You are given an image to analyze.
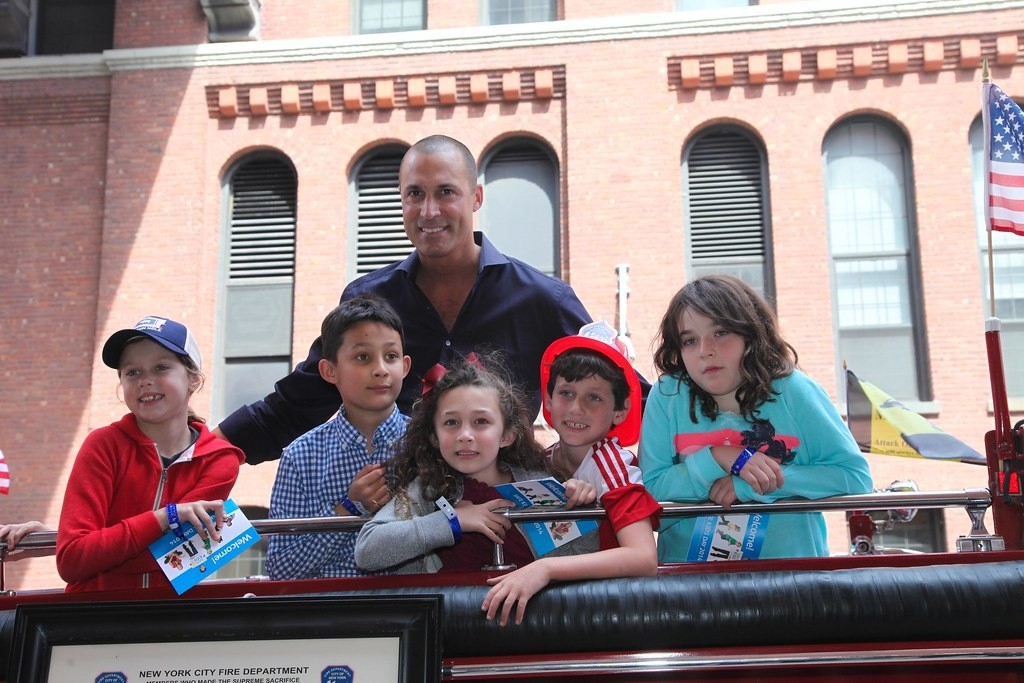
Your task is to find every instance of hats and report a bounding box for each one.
[542,321,644,451]
[102,317,204,375]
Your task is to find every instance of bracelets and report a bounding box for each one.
[165,503,185,539]
[340,493,371,518]
[434,495,464,545]
[730,445,758,476]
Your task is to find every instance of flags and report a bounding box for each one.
[844,369,989,467]
[981,81,1024,235]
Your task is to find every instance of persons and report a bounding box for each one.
[481,318,664,625]
[265,294,413,583]
[0,134,653,563]
[55,315,244,593]
[637,273,874,564]
[353,350,599,576]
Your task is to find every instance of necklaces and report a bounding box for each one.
[708,413,741,446]
